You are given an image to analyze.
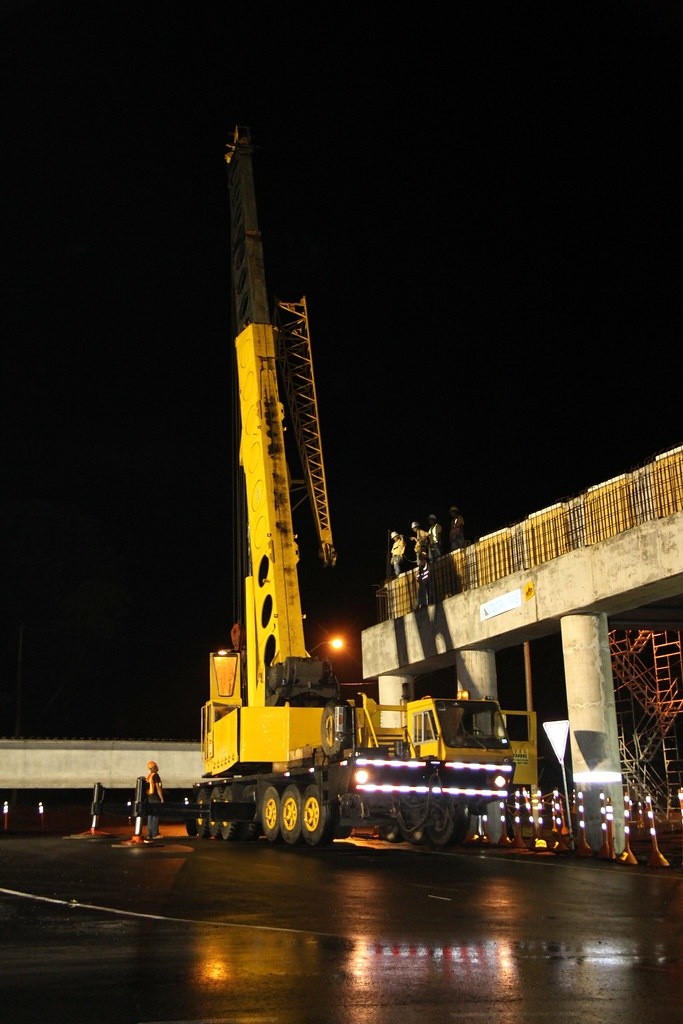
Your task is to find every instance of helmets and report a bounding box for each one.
[450,506,458,511]
[148,761,156,770]
[391,531,398,539]
[411,522,419,529]
[429,514,436,519]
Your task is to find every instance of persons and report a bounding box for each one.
[449,508,465,550]
[411,551,431,610]
[390,531,406,577]
[147,761,164,839]
[410,521,429,565]
[428,513,443,559]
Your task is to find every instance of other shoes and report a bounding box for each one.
[147,833,164,838]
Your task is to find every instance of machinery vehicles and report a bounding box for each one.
[179,119,542,845]
